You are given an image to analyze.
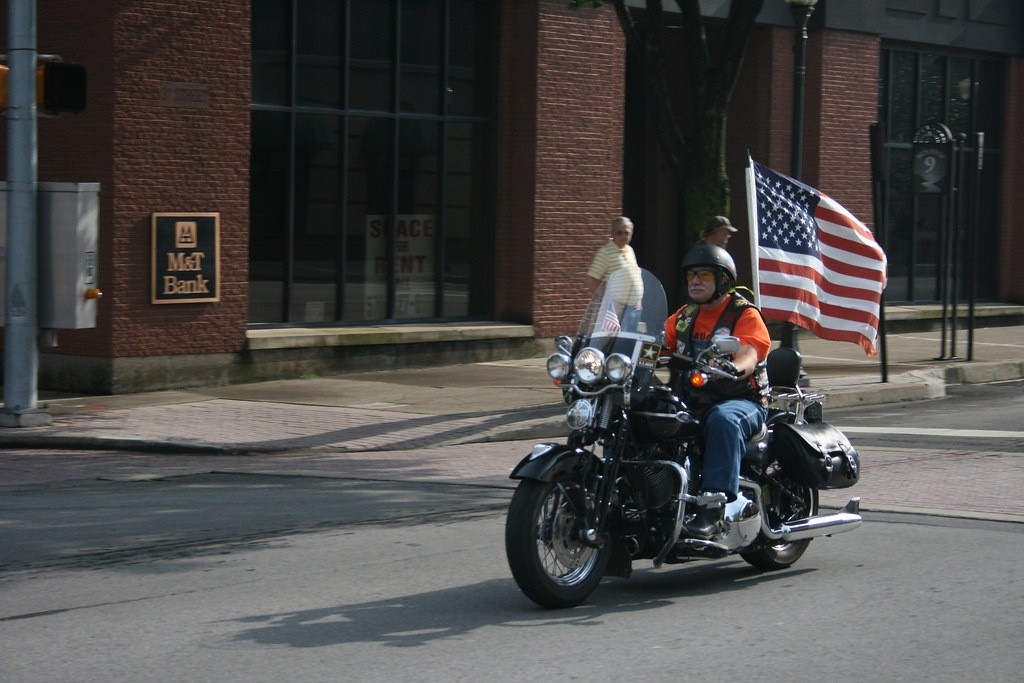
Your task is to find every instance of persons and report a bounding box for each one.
[587,217,644,332]
[701,215,737,247]
[657,244,771,541]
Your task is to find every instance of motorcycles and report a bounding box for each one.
[504,267,862,609]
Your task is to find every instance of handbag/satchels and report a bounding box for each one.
[773,419,860,489]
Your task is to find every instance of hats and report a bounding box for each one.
[681,245,736,287]
[708,216,737,232]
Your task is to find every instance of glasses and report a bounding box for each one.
[685,269,714,281]
[615,230,633,235]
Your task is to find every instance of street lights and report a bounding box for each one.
[779,0,818,381]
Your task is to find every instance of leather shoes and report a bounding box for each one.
[686,504,727,539]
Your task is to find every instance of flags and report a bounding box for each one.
[603,302,622,332]
[750,159,888,358]
